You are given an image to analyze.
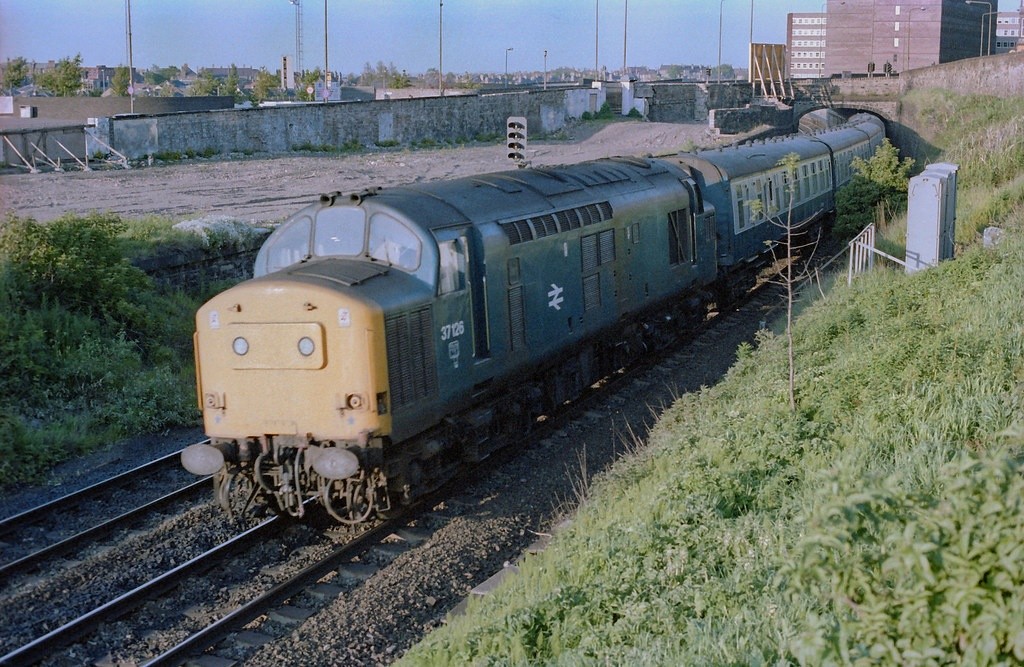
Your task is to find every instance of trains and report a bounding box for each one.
[178,111,887,528]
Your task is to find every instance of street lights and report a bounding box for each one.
[505,46,513,93]
